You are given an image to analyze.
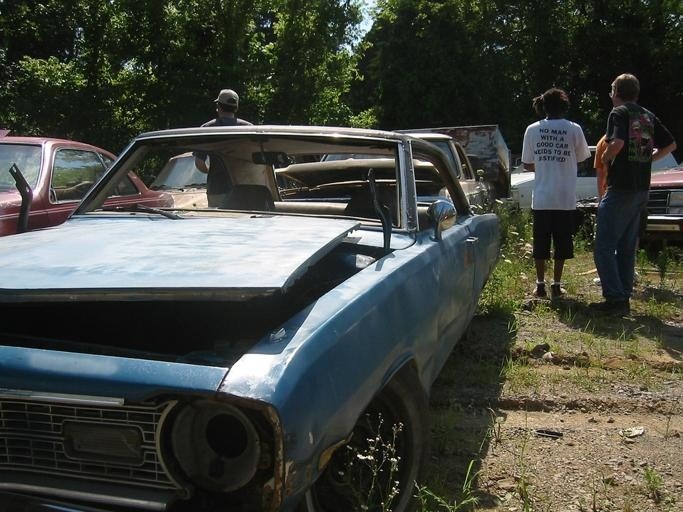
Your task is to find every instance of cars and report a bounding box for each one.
[503,145,683,233]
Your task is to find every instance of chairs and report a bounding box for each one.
[219,183,400,221]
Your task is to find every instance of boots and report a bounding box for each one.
[533,279,548,299]
[550,282,568,301]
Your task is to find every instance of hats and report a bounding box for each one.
[212,88,239,107]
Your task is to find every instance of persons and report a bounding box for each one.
[519,88,593,299]
[190,88,262,209]
[592,107,642,286]
[583,73,678,315]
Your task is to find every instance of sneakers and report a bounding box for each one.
[588,299,630,316]
